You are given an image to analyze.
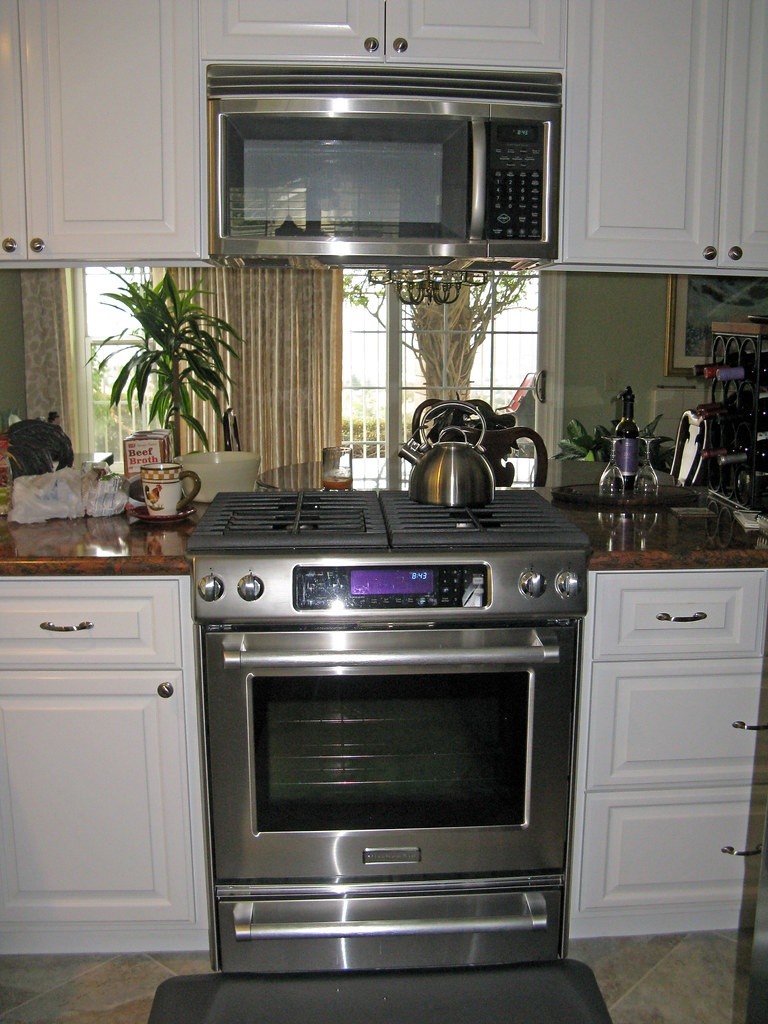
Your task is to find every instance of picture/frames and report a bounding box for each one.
[664,273,768,377]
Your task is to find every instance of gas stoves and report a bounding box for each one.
[183,489,590,624]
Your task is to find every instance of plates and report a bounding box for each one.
[174,451,262,503]
[126,507,196,523]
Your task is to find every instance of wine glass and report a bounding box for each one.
[599,436,626,499]
[634,436,661,496]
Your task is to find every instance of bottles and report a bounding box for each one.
[613,386,639,489]
[703,350,768,379]
[702,397,768,429]
[702,432,768,459]
[717,439,768,474]
[697,390,768,417]
[0,440,12,514]
[716,352,768,387]
[693,349,768,376]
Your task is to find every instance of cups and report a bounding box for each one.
[140,463,202,515]
[322,447,352,490]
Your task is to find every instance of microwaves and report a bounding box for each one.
[208,65,564,272]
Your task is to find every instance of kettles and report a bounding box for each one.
[398,401,496,508]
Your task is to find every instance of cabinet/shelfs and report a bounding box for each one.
[0,0,768,279]
[0,573,209,955]
[565,570,768,936]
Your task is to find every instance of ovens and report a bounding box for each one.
[202,626,582,974]
